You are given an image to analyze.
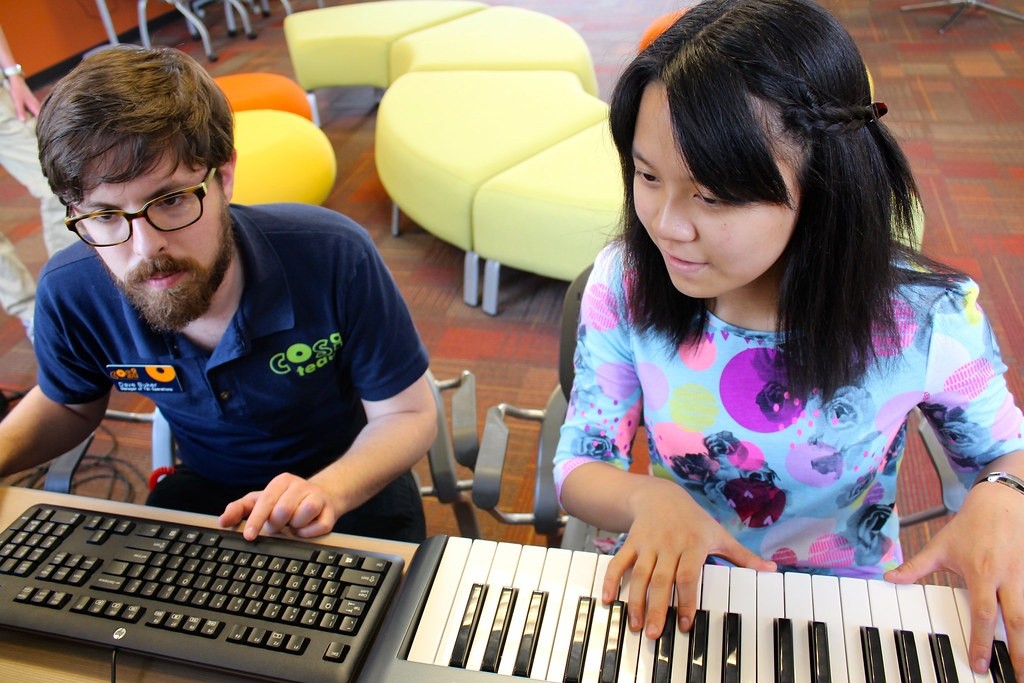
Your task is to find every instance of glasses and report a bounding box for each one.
[65,163,219,246]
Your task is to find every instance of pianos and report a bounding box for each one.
[353,531,1016,683]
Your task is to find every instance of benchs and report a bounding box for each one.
[283,1,631,317]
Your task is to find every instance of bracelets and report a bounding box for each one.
[969,471,1023,496]
[2,63,25,79]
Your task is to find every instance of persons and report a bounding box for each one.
[0,43,438,543]
[554,0,1023,682]
[0,18,85,346]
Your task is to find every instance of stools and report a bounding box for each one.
[211,71,337,206]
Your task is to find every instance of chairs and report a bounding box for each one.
[471,263,970,555]
[43,367,479,539]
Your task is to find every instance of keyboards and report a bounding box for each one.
[0,504,404,683]
